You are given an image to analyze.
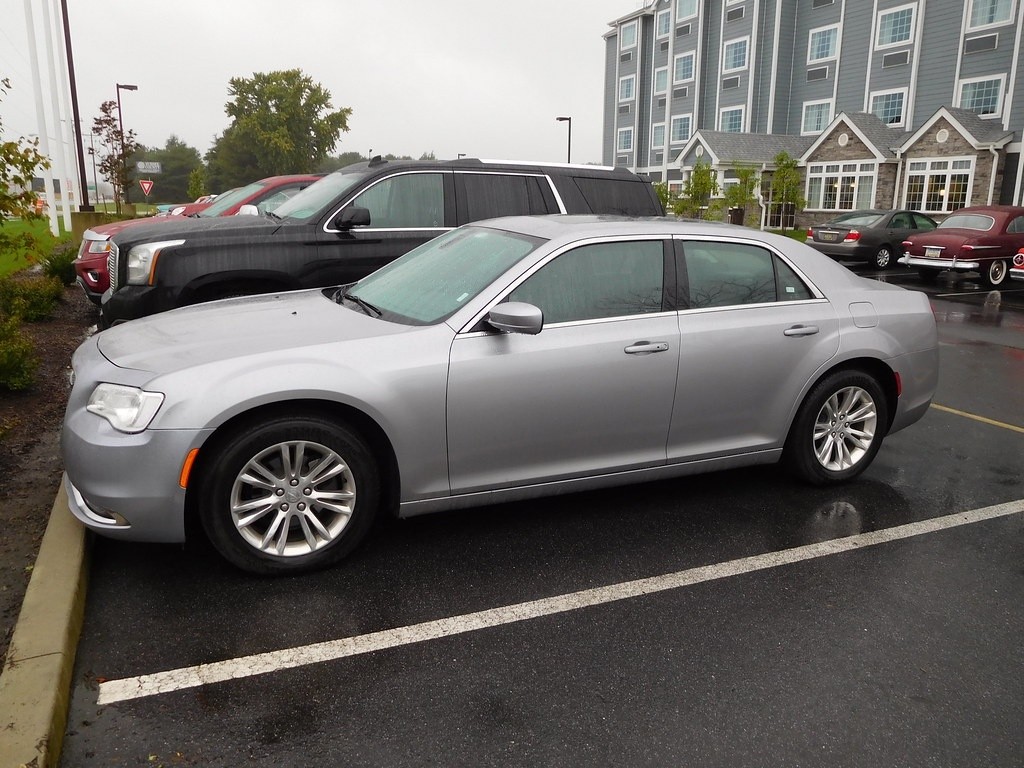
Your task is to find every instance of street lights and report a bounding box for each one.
[556,116,571,163]
[116,83,138,203]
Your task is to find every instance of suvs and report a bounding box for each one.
[98,158,667,330]
[72,172,326,307]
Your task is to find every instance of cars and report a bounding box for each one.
[1010,247,1024,281]
[805,209,939,269]
[896,205,1024,290]
[60,213,941,575]
